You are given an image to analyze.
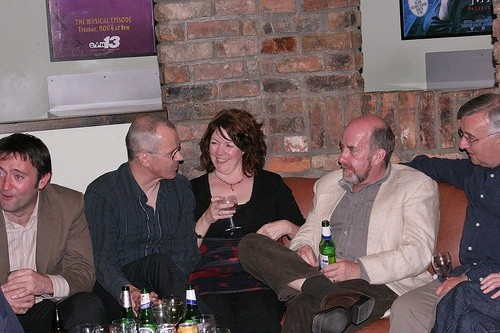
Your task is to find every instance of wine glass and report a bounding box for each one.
[218,195,242,231]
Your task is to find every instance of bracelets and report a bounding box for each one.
[195,231,204,239]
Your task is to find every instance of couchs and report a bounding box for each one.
[280,177,468,333]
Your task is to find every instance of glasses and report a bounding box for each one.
[457,127,497,145]
[149,146,181,160]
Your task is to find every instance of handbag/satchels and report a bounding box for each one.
[188,234,292,296]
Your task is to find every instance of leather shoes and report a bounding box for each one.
[310,286,375,333]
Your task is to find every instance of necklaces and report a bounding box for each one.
[213,171,244,191]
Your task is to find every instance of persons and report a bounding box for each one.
[240,117,440,333]
[188,109,306,333]
[431,272,500,333]
[85,113,216,333]
[389,94,500,333]
[0,134,105,333]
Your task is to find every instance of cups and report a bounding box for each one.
[178,323,197,333]
[192,314,230,333]
[431,252,452,277]
[151,295,184,333]
[75,322,101,333]
[111,318,136,333]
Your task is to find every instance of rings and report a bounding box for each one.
[332,277,335,282]
[218,210,221,216]
[217,201,220,204]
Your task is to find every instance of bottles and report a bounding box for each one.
[51,308,63,333]
[137,287,157,333]
[319,220,336,270]
[119,286,138,333]
[180,284,202,323]
